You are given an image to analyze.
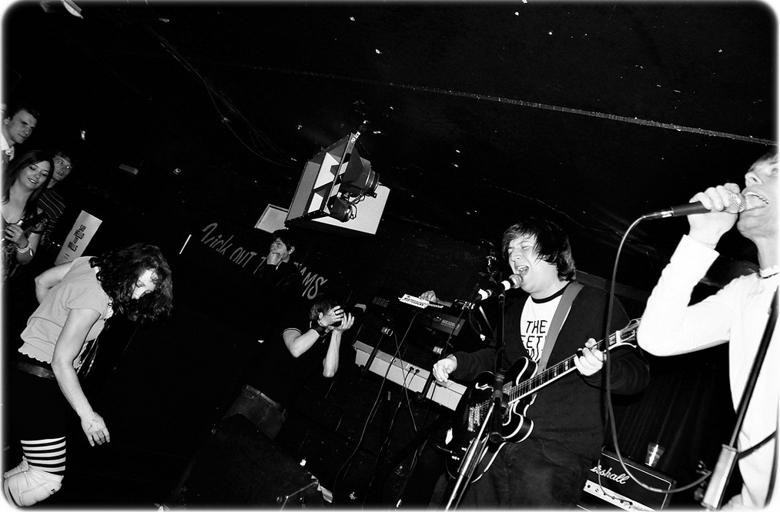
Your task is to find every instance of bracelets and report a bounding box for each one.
[312,320,329,336]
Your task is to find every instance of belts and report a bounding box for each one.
[16,360,54,379]
[244,384,288,417]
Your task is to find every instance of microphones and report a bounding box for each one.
[654,192,747,219]
[475,274,523,302]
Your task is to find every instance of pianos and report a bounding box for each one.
[352,340,467,412]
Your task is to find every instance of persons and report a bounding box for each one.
[428,216,651,510]
[635,144,779,510]
[156,293,358,509]
[253,229,303,297]
[5,242,176,509]
[0,100,77,292]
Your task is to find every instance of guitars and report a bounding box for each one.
[444,316,640,481]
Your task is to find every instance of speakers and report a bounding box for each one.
[575,450,678,512]
